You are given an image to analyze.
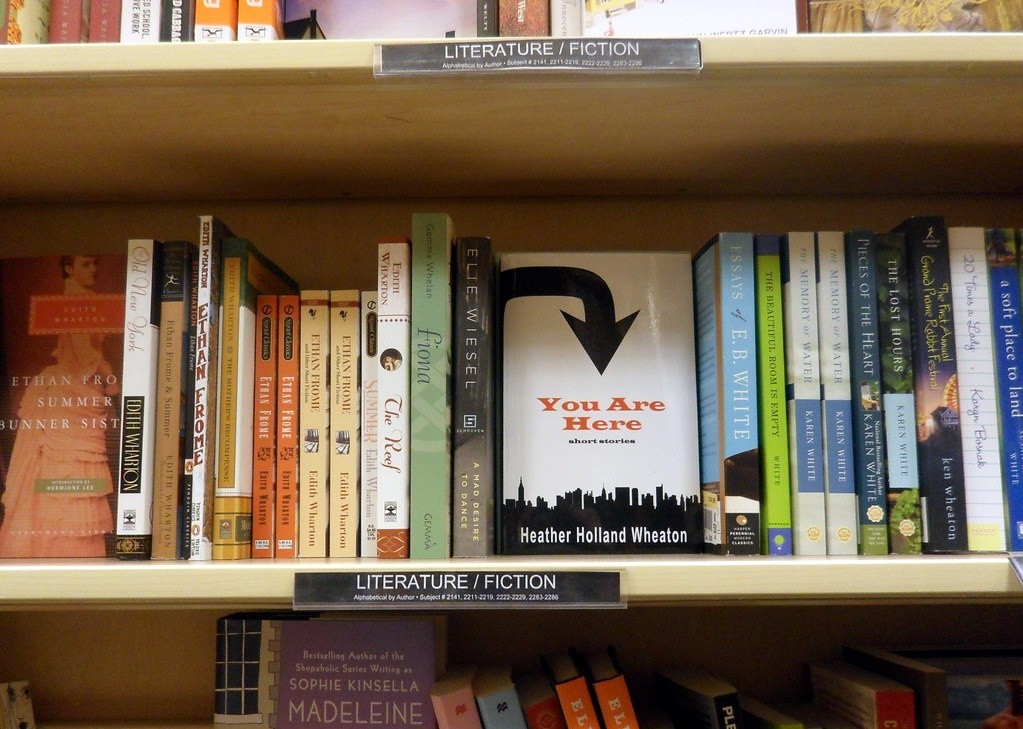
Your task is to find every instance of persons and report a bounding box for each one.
[0,257,121,557]
[383,348,401,371]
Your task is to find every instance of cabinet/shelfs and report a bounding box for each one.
[0,32,1022,729]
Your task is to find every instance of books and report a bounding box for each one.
[274,612,1023,729]
[0,213,1023,561]
[3,0,1023,45]
